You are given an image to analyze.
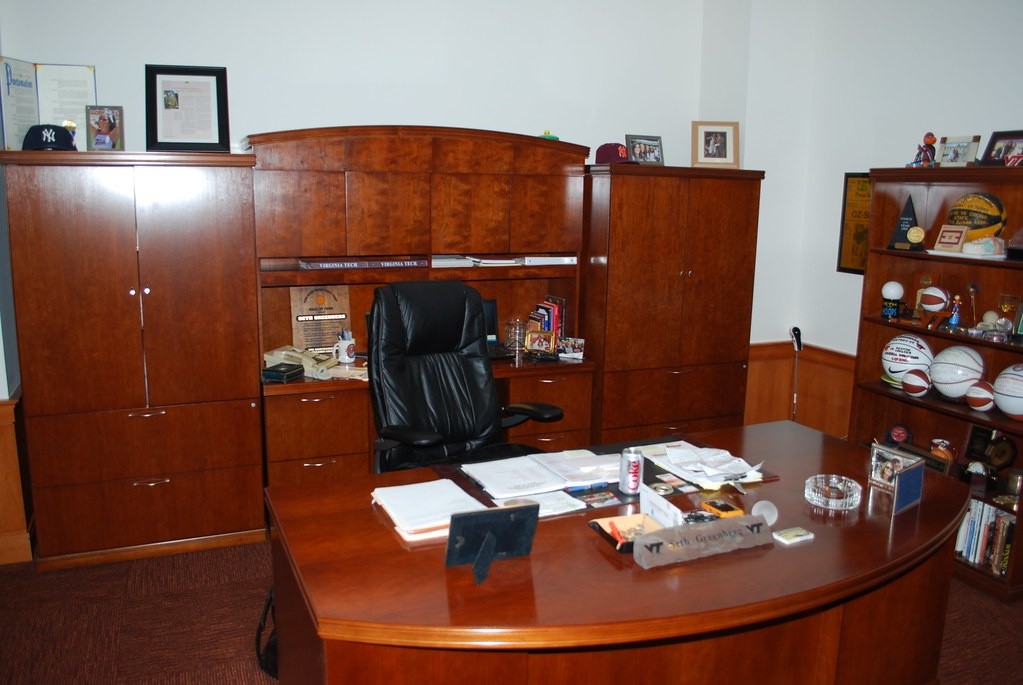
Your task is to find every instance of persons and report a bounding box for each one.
[947,149,959,162]
[879,458,904,484]
[89,112,120,148]
[992,140,1016,159]
[632,142,660,162]
[704,132,725,158]
[556,339,583,354]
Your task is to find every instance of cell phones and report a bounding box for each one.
[701,499,744,519]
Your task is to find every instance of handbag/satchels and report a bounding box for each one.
[255,587,279,678]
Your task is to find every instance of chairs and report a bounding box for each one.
[366,278,565,473]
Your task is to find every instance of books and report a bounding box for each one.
[459,449,620,501]
[953,499,1017,577]
[526,294,565,355]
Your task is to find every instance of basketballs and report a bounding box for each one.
[993,363,1023,422]
[882,333,934,383]
[931,345,989,402]
[947,192,1007,242]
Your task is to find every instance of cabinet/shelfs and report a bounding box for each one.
[587,164,766,439]
[849,165,1023,606]
[1,151,267,572]
[244,124,597,483]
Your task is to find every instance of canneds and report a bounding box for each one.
[618,448,644,495]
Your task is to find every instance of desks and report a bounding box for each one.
[261,417,975,684]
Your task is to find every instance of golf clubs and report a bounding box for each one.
[790,326,804,421]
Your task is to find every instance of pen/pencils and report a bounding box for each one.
[564,481,609,492]
[335,327,353,342]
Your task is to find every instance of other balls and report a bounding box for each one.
[967,382,995,413]
[920,286,950,312]
[902,369,932,398]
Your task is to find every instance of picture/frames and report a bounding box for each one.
[624,132,665,166]
[932,141,979,164]
[981,130,1023,165]
[868,440,924,494]
[837,173,880,275]
[522,329,556,355]
[85,103,125,153]
[143,63,231,153]
[690,118,741,170]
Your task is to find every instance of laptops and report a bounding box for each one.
[481,300,516,359]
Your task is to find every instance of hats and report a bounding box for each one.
[22,125,77,151]
